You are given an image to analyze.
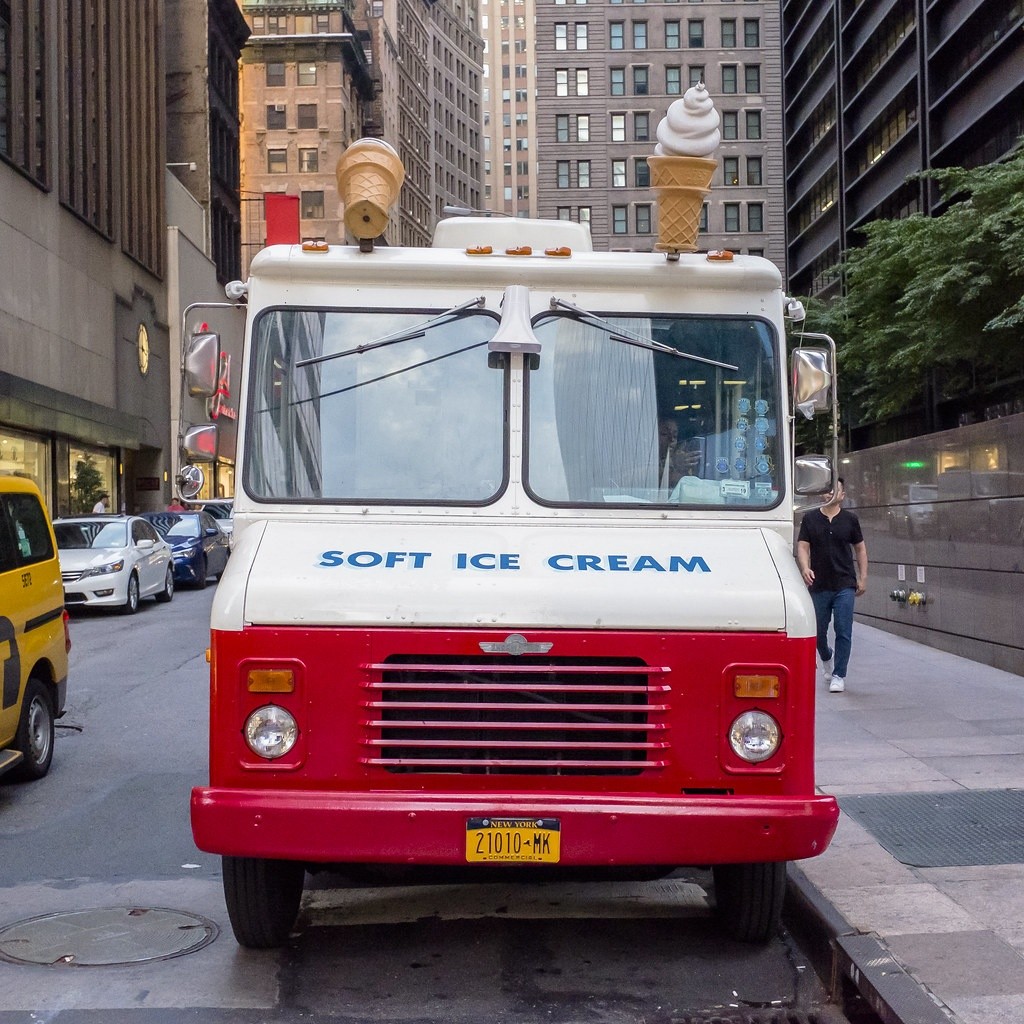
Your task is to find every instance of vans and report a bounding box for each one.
[0,474,71,779]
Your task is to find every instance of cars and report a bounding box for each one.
[51,513,176,615]
[138,497,234,589]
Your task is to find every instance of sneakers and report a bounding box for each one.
[829,676,845,692]
[822,650,834,679]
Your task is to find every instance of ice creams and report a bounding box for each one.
[645,80,721,253]
[335,137,404,239]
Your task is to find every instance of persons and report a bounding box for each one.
[797,477,868,692]
[167,498,185,511]
[658,417,703,490]
[92,494,108,513]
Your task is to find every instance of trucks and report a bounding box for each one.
[175,80,838,948]
